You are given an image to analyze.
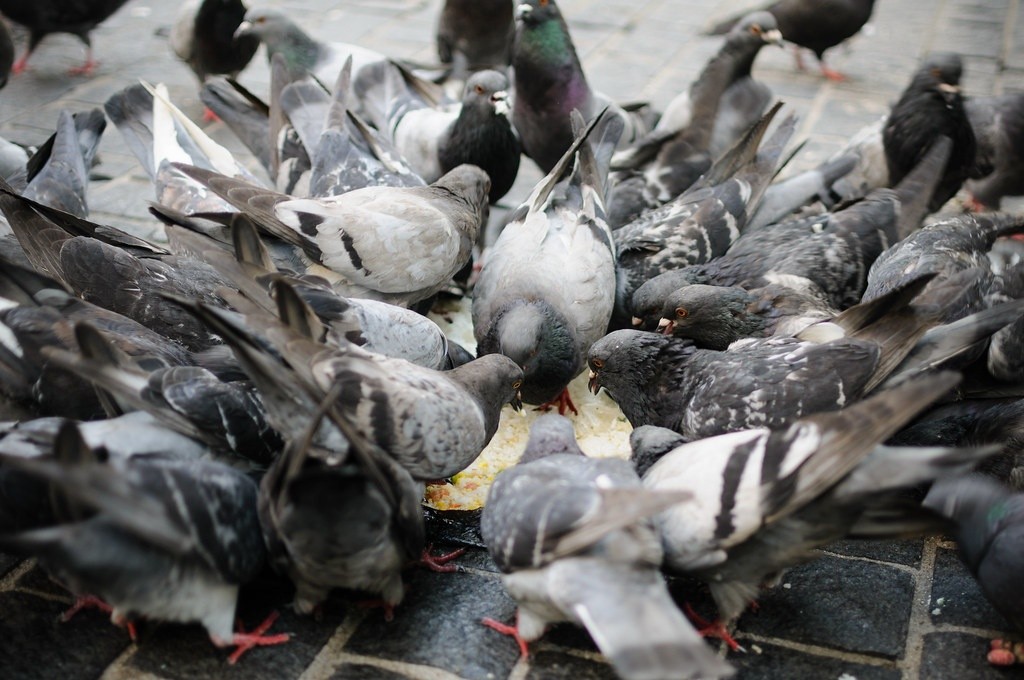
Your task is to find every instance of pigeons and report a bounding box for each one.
[0,0,1021,679]
